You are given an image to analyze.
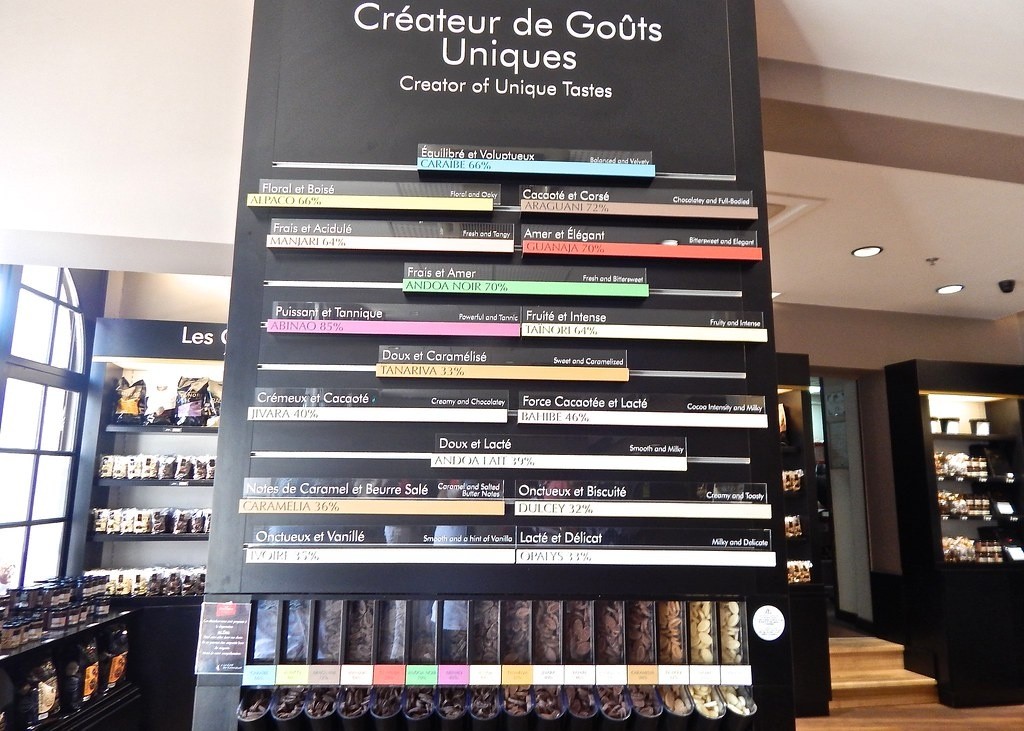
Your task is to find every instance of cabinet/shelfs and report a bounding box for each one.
[65,317,228,731]
[0,606,141,731]
[776,352,830,718]
[884,358,1024,709]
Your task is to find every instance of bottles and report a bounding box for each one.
[0,575,110,649]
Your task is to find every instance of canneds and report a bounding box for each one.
[0,575,110,651]
